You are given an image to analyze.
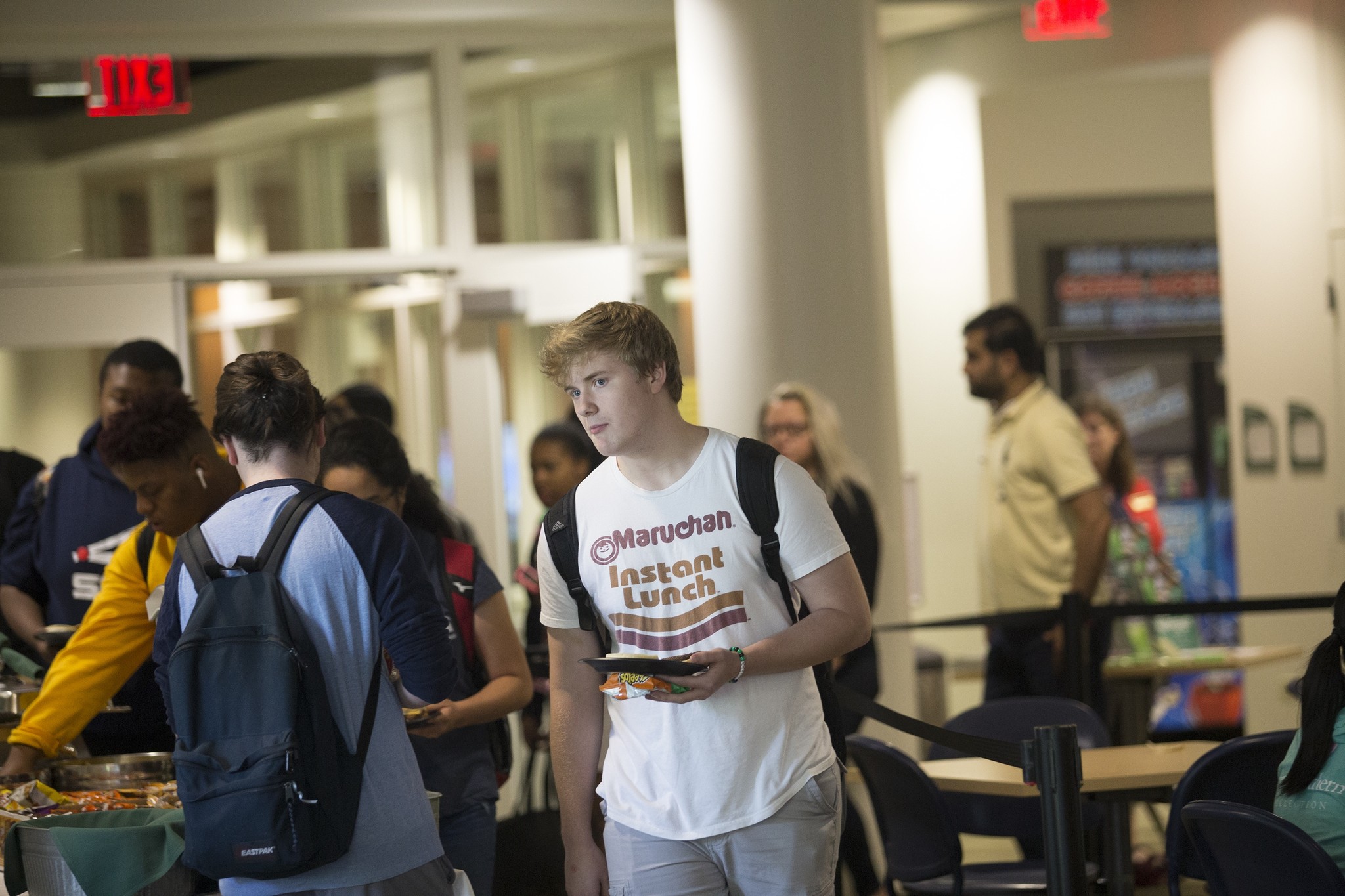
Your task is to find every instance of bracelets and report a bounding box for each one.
[724,647,747,683]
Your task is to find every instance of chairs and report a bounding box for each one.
[836,694,1345,896]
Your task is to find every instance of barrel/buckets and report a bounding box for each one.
[15,809,194,896]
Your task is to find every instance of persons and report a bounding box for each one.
[521,303,873,896]
[964,304,1108,860]
[1272,584,1345,876]
[0,340,879,896]
[1056,402,1180,892]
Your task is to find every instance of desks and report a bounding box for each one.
[953,645,1302,732]
[923,737,1231,874]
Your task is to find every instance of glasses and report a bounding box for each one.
[765,423,807,436]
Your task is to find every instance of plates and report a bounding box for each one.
[404,709,443,728]
[572,657,706,676]
[32,631,76,639]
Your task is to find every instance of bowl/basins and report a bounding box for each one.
[0,676,45,715]
[39,752,178,791]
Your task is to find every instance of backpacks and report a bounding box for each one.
[166,488,383,881]
[442,538,513,789]
